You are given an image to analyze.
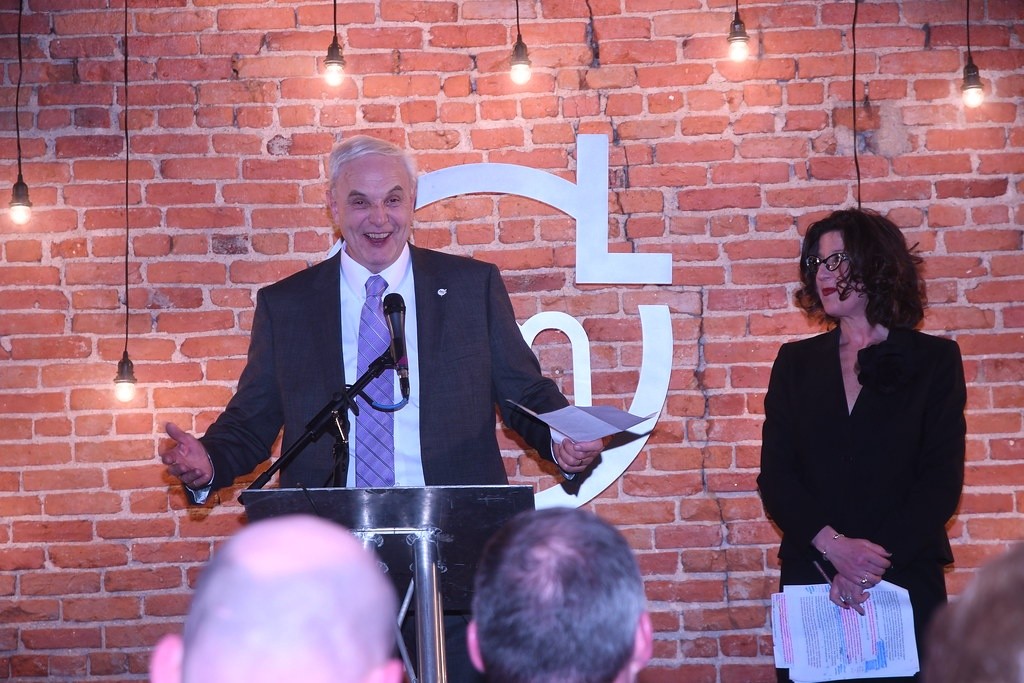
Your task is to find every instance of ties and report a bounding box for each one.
[355,275,395,489]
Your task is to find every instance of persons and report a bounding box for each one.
[925,544,1024,683]
[465,503,654,683]
[162,135,616,683]
[756,207,966,683]
[147,513,406,683]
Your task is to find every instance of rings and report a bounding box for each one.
[578,460,583,466]
[843,595,852,600]
[861,575,868,584]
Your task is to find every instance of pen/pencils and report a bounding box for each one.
[814,561,849,607]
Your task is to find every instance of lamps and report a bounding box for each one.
[113,0,139,403]
[8,0,34,223]
[728,0,752,64]
[323,0,349,87]
[507,0,534,85]
[959,0,985,109]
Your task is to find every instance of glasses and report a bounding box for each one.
[806,253,850,272]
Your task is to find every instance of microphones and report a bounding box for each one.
[383,293,409,401]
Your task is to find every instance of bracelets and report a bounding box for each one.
[821,533,844,562]
